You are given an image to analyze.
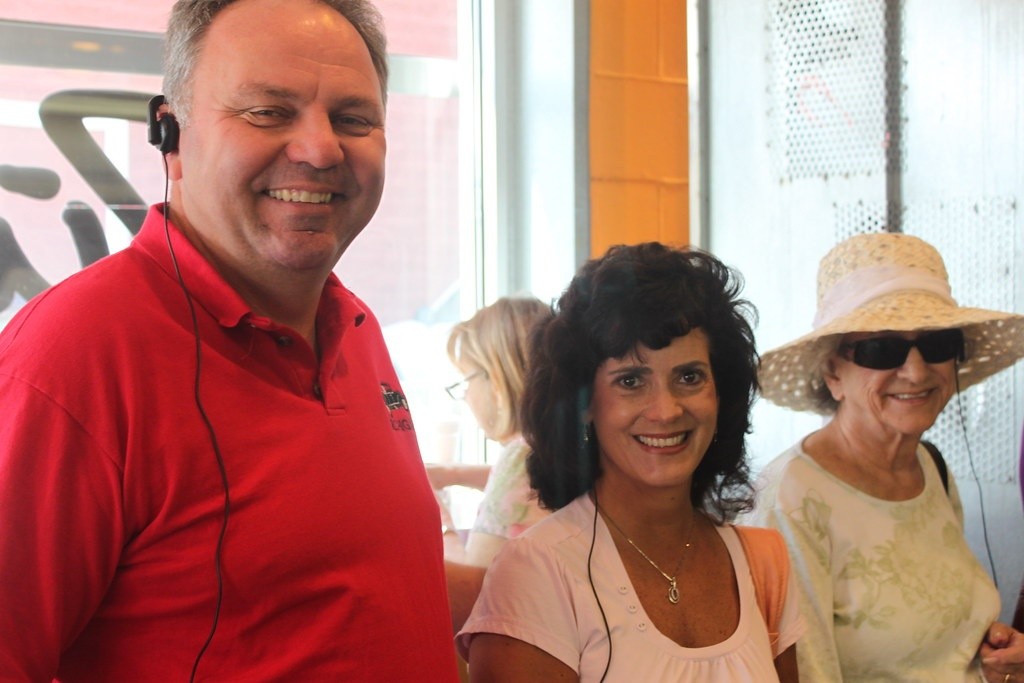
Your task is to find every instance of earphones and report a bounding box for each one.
[146,95,179,153]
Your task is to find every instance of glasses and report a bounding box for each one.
[836,329,964,369]
[444,373,480,399]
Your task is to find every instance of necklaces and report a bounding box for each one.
[595,499,697,605]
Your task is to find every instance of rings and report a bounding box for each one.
[1004,673,1011,683]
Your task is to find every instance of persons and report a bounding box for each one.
[454,239,803,683]
[734,232,1024,683]
[431,295,554,640]
[0,0,485,683]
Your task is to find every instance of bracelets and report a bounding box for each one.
[440,523,458,535]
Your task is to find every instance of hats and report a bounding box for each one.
[753,234,1024,417]
